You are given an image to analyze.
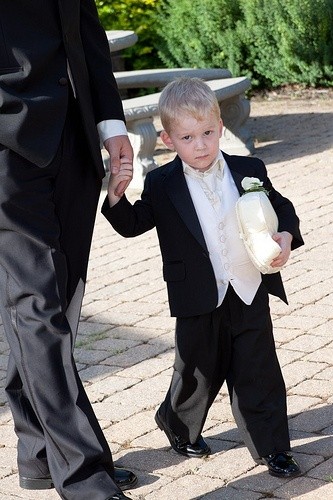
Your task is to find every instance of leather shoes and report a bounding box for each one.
[18,466,139,491]
[255,449,302,478]
[108,493,134,500]
[153,409,212,458]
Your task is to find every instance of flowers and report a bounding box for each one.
[240,176,272,196]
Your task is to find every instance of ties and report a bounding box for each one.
[186,163,222,212]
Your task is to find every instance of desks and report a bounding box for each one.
[104,28,139,72]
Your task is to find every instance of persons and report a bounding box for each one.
[0,0,140,500]
[101,77,304,477]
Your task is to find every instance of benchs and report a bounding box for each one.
[111,67,255,177]
